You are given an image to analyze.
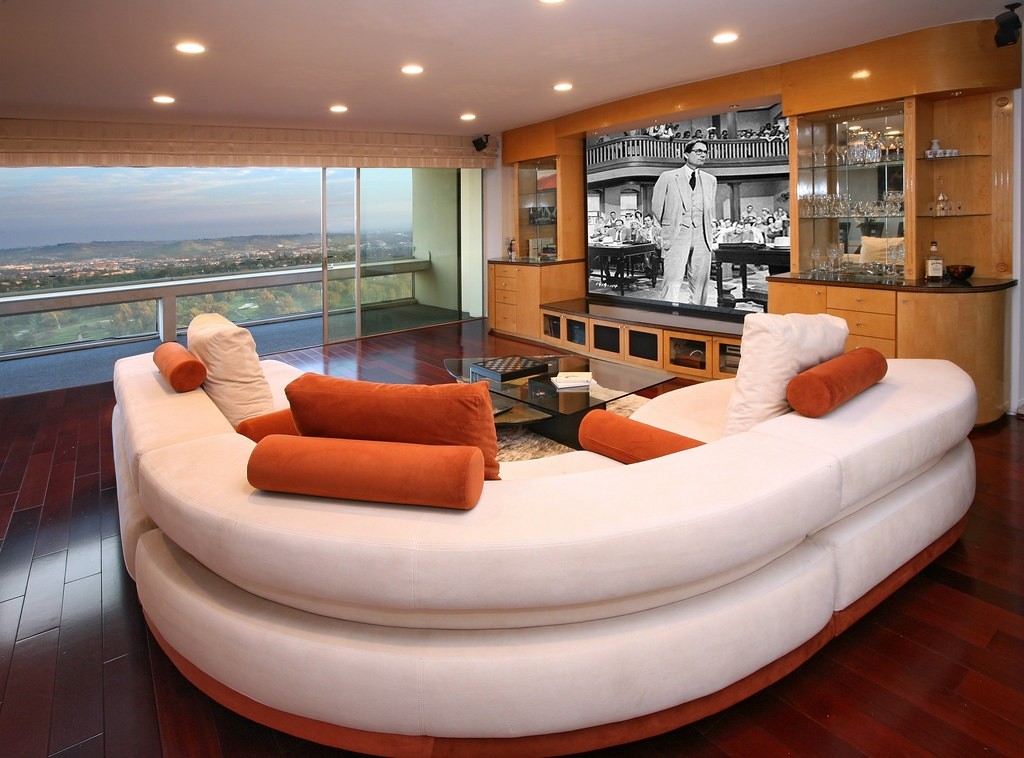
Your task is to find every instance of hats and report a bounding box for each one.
[625,213,633,216]
[707,127,716,132]
[762,208,769,213]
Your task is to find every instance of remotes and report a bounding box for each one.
[493,405,512,416]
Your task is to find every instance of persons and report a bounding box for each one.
[652,140,718,305]
[590,205,790,271]
[594,122,790,162]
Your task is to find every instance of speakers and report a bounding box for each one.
[473,137,487,151]
[995,18,1020,47]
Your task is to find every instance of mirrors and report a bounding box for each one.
[812,108,905,274]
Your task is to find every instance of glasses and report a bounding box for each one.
[691,149,707,155]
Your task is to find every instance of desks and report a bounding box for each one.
[587,240,790,308]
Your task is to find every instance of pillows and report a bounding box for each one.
[153,313,502,510]
[580,409,706,466]
[723,311,850,439]
[860,236,906,264]
[786,347,888,419]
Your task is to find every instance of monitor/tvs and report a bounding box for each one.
[585,102,793,322]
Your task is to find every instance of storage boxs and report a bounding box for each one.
[675,351,705,369]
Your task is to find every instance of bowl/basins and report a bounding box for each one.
[946,264,974,281]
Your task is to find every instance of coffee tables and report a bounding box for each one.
[443,354,676,442]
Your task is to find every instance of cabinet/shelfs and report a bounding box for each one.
[765,86,1016,429]
[487,154,587,342]
[540,298,744,381]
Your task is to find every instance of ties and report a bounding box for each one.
[690,172,696,190]
[618,231,620,240]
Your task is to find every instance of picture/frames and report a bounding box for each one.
[878,148,903,211]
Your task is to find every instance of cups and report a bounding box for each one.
[928,201,961,215]
[924,149,959,158]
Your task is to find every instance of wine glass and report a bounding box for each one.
[806,143,846,166]
[881,136,904,162]
[802,190,903,216]
[810,242,845,272]
[889,240,905,276]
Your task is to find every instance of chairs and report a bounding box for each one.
[839,221,883,254]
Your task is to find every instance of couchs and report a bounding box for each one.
[494,378,651,459]
[111,348,978,758]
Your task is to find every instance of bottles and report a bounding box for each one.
[925,241,943,281]
[931,139,940,149]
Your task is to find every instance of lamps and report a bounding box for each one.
[473,135,491,152]
[994,2,1022,46]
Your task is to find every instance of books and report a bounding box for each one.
[550,372,594,389]
